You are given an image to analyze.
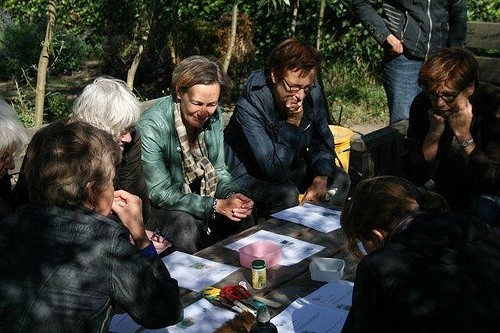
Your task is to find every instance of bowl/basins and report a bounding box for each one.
[239,240,282,269]
[310,258,345,281]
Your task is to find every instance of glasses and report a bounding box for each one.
[279,74,316,92]
[429,89,463,102]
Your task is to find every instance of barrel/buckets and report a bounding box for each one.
[329,125,353,172]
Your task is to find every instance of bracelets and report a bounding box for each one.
[210,198,217,221]
[226,192,236,199]
[461,136,474,147]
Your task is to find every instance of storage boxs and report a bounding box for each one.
[239,240,282,269]
[309,257,346,282]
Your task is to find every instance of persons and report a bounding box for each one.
[0,120,184,333]
[353,0,467,124]
[0,98,27,202]
[228,38,353,220]
[341,175,500,333]
[117,55,256,255]
[67,75,151,227]
[407,46,500,229]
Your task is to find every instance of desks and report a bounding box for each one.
[102,193,360,333]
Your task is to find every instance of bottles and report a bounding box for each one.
[252,260,266,289]
[249,305,278,333]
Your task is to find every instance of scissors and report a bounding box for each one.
[225,285,262,311]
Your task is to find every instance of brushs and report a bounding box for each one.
[215,309,256,333]
